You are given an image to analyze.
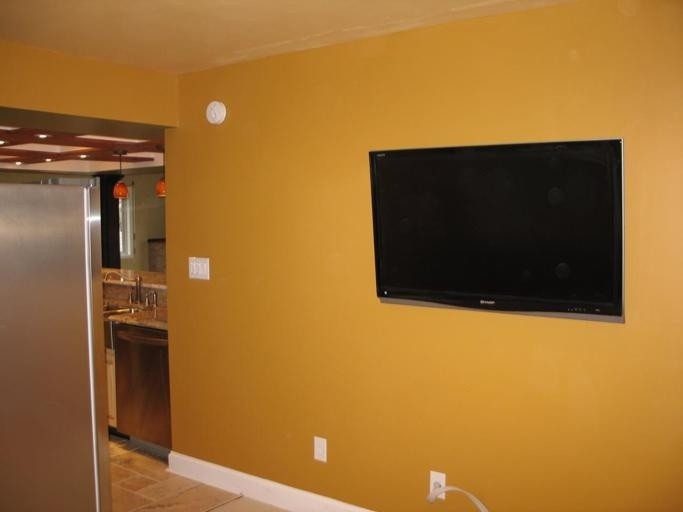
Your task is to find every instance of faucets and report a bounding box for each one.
[104,272,142,305]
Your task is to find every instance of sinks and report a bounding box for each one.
[103,304,145,319]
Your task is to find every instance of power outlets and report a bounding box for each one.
[429,470,446,499]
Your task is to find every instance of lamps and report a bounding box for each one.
[112,149,129,200]
[154,145,168,198]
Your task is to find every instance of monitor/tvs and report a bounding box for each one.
[367,137,625,323]
